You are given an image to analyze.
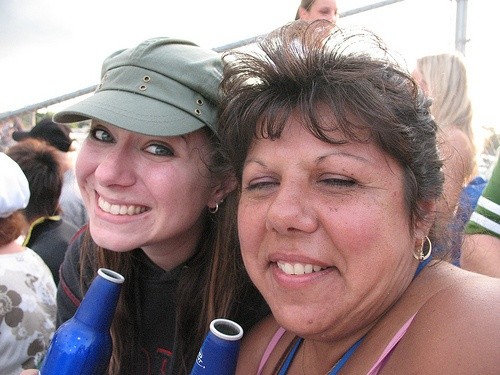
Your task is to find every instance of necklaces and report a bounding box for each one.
[278,256,434,375]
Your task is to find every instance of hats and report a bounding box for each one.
[0,152,30,218]
[54,37,249,136]
[12,119,72,152]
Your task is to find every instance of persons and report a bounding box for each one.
[19,37,270,375]
[12,117,89,229]
[6,140,81,288]
[215,18,500,375]
[293,0,337,60]
[410,53,500,278]
[0,151,58,375]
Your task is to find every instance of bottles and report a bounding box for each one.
[189,319,244,375]
[39,268,125,375]
